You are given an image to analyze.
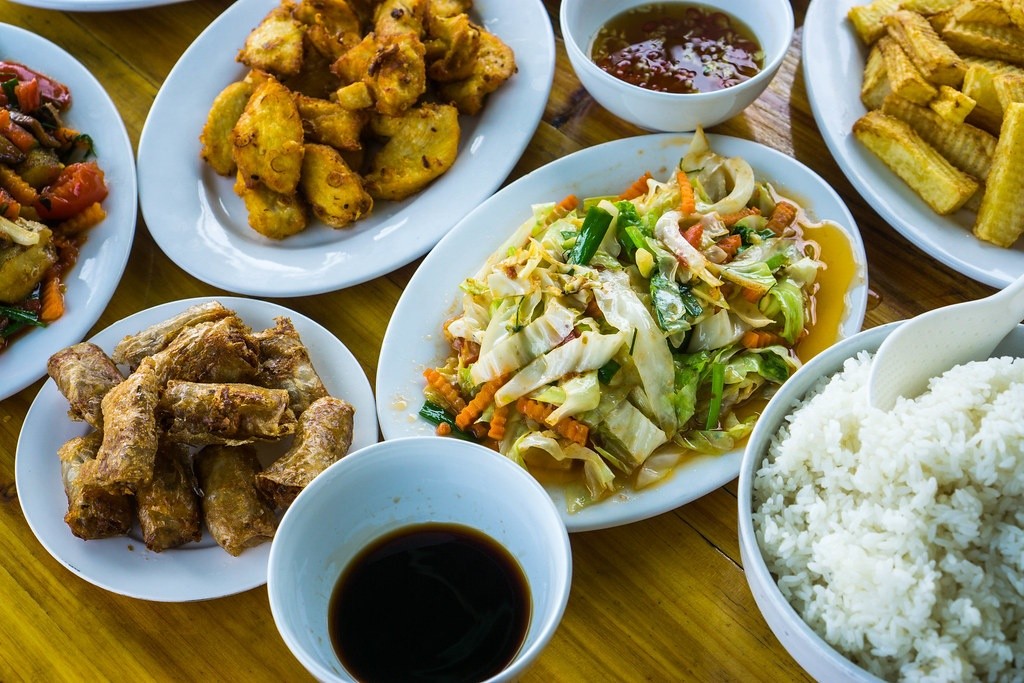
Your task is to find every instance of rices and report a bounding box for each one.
[754,347,1024,683]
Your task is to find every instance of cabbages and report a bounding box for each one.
[449,155,820,508]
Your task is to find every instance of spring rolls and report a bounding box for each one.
[48,304,354,559]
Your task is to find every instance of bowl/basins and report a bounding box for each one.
[561,0,794,131]
[267,437,571,683]
[738,317,1024,683]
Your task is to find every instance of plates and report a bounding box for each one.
[14,298,377,601]
[804,0,1024,290]
[137,0,555,297]
[376,130,867,532]
[0,24,136,403]
